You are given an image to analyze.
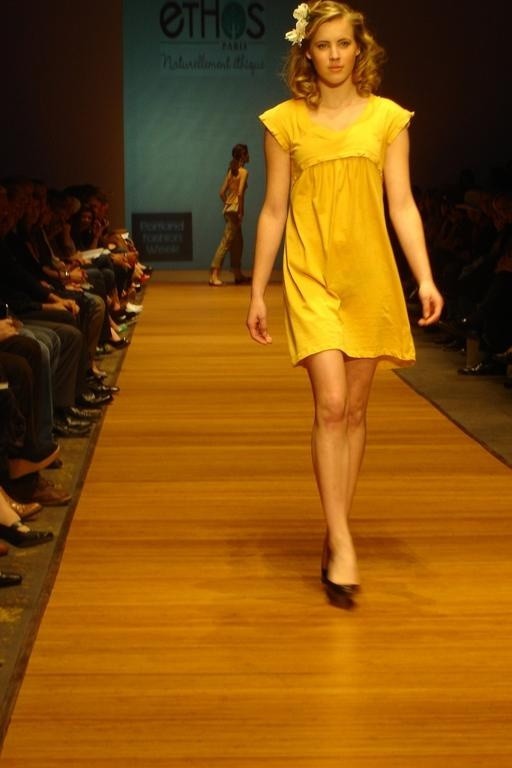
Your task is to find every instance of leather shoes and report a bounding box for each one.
[50,367,119,435]
[433,333,467,351]
[93,301,143,355]
[0,443,70,583]
[127,265,153,298]
[458,356,506,375]
[321,568,359,596]
[491,350,512,365]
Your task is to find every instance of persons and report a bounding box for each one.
[0,176,156,596]
[208,140,251,286]
[398,160,512,391]
[242,3,449,602]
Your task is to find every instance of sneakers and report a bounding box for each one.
[235,275,252,284]
[209,276,222,285]
